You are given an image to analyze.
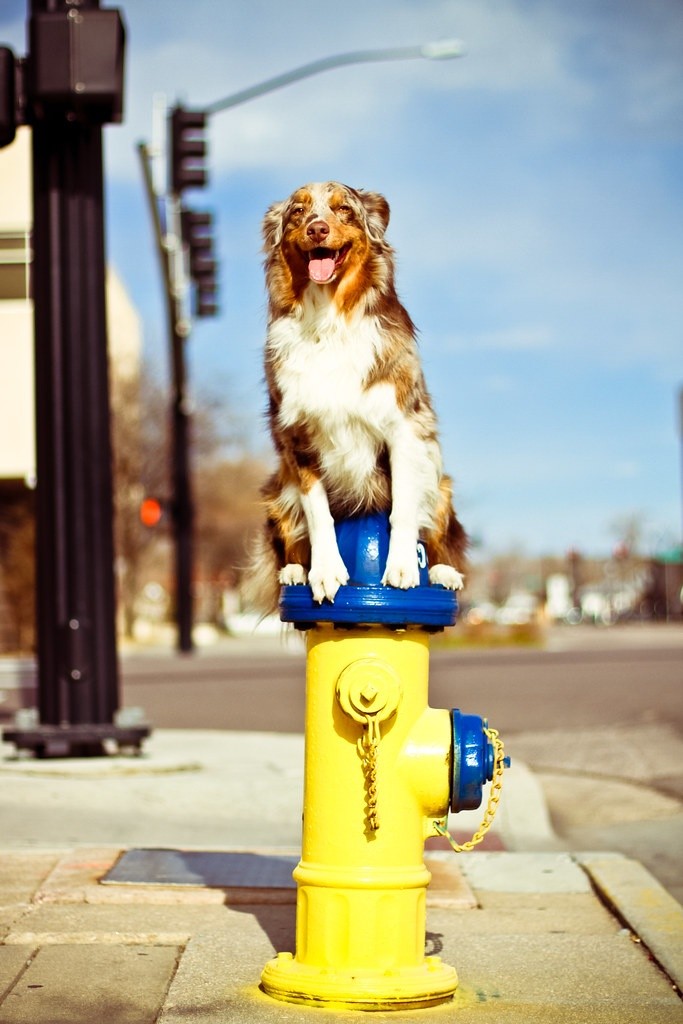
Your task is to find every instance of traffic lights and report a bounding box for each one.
[139,497,169,530]
[169,103,207,196]
[23,7,129,126]
[180,211,215,316]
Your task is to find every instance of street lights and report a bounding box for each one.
[135,41,468,649]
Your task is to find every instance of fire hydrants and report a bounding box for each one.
[259,510,512,1015]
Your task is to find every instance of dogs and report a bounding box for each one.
[231,180,474,651]
